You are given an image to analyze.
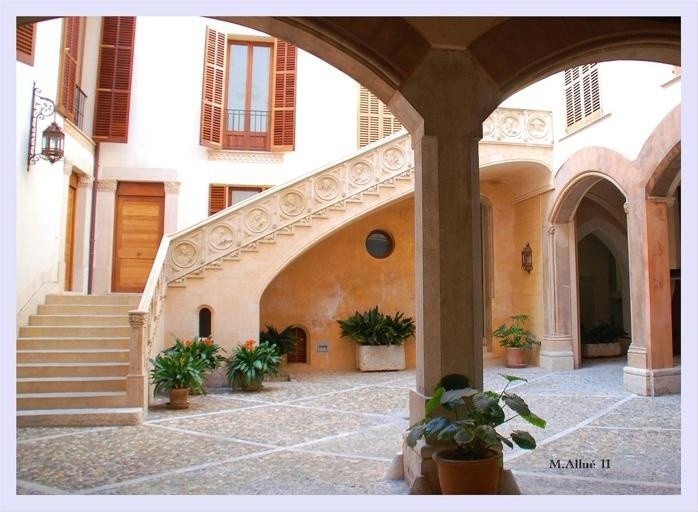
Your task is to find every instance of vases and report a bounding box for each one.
[237,369,264,392]
[187,377,200,395]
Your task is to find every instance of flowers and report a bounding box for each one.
[175,335,229,373]
[224,338,285,393]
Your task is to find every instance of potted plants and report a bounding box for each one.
[404,373,545,495]
[491,315,540,368]
[259,321,299,374]
[581,314,630,359]
[335,304,416,372]
[148,346,206,409]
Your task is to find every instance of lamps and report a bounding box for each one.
[520,242,533,275]
[27,81,65,173]
